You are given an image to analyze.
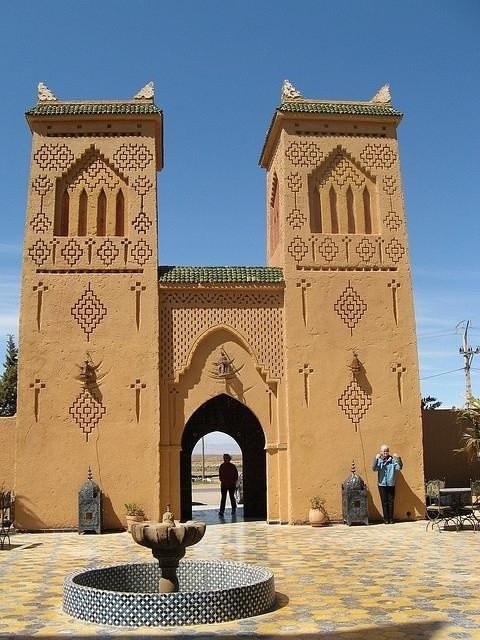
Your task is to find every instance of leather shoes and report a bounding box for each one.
[385,521,394,524]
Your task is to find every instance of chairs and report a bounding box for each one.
[424,476,480,535]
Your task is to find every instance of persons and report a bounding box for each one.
[371,444,403,523]
[217,453,239,516]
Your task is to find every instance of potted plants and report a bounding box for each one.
[124,502,146,532]
[308,495,329,527]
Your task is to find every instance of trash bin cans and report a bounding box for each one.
[341,457,369,527]
[78,464,101,535]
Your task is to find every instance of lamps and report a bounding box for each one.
[349,350,361,374]
[216,346,233,378]
[81,361,94,379]
